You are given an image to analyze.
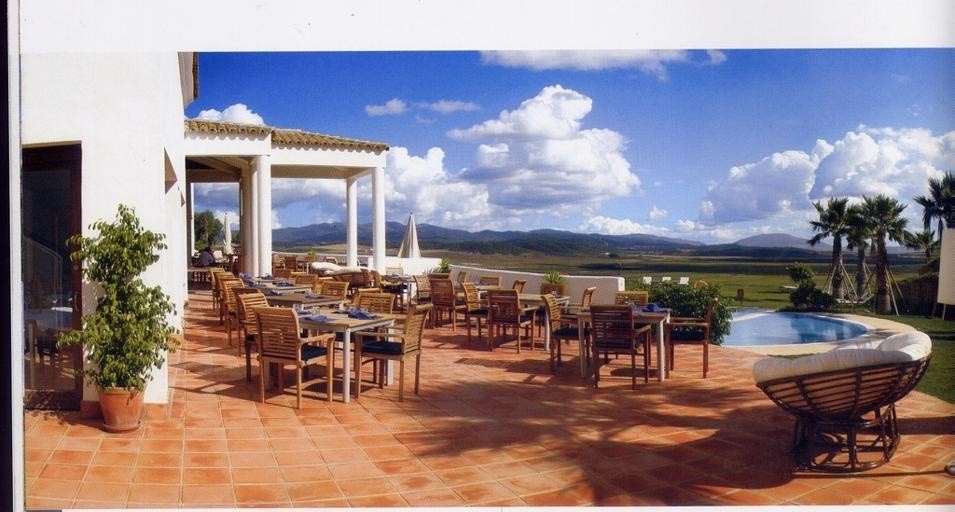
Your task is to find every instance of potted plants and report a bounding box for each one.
[56,201,183,427]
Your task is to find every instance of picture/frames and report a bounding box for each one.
[22,234,64,331]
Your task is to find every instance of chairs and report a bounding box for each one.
[460,281,487,344]
[486,289,539,354]
[479,276,501,285]
[560,285,598,345]
[190,254,468,410]
[835,292,875,312]
[664,298,718,379]
[590,303,651,390]
[642,276,690,286]
[512,279,527,293]
[614,290,651,367]
[540,293,579,375]
[751,331,932,473]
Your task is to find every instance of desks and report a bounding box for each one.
[576,309,672,382]
[513,292,571,352]
[416,286,503,331]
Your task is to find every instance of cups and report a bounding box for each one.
[272,302,369,317]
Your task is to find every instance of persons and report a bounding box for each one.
[199,247,213,266]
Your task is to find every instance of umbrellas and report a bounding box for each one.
[222,212,232,255]
[398,211,421,258]
